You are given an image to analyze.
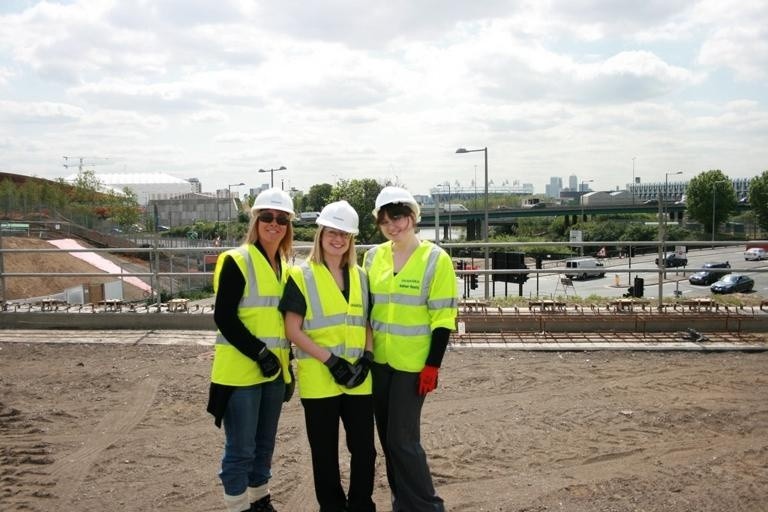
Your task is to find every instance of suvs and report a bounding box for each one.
[688,261,731,287]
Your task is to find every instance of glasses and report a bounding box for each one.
[259,212,289,225]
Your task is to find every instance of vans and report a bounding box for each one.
[293,210,323,228]
[445,204,470,214]
[565,259,606,280]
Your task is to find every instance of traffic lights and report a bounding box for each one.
[470,273,478,290]
[522,265,530,283]
[456,260,463,278]
[635,278,645,298]
[534,255,542,269]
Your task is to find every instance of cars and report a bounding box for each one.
[495,206,513,211]
[710,274,755,295]
[667,201,686,206]
[655,254,687,267]
[530,202,567,209]
[737,196,752,205]
[744,247,768,261]
[109,224,170,234]
[641,198,659,206]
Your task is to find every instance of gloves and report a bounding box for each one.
[418,366,438,395]
[257,348,281,377]
[323,351,374,390]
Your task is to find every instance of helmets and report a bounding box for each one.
[316,200,360,235]
[373,186,421,223]
[252,189,295,216]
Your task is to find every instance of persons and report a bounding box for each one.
[207,187,296,512]
[362,186,458,512]
[278,200,376,512]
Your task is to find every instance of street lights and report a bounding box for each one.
[580,178,595,256]
[754,213,760,239]
[663,171,683,279]
[258,165,288,187]
[225,182,246,246]
[711,179,726,249]
[631,154,637,207]
[436,183,452,261]
[474,163,477,210]
[456,147,490,299]
